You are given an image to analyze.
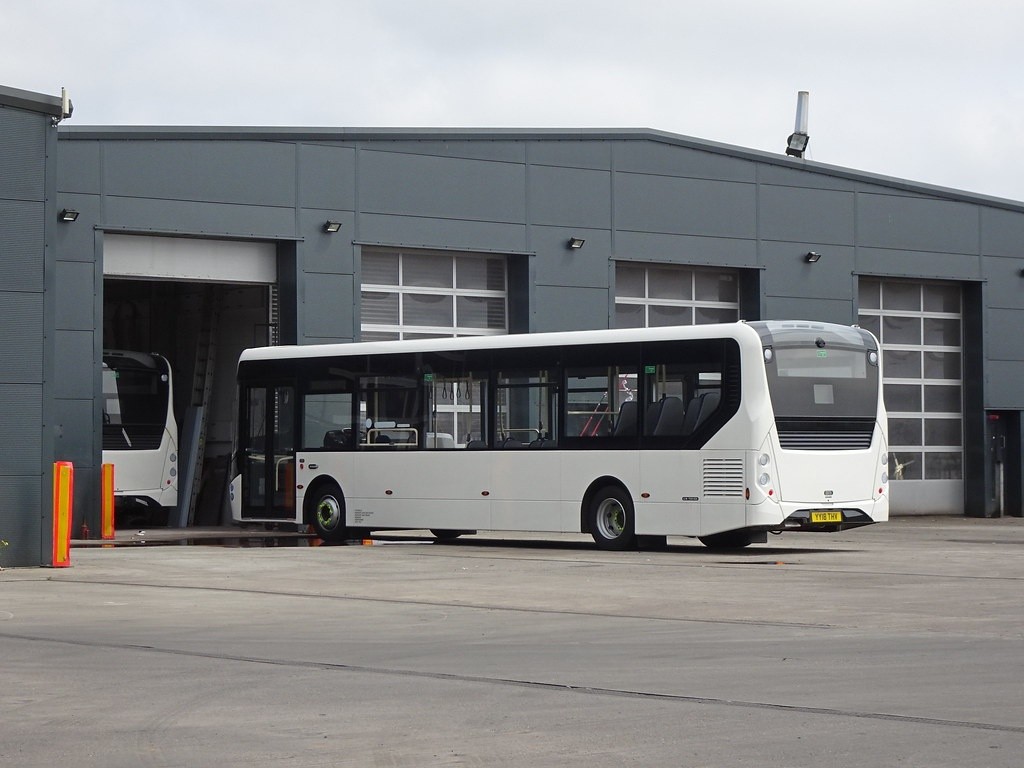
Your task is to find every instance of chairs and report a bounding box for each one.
[694,392,721,432]
[679,397,705,435]
[612,401,638,437]
[466,437,556,449]
[647,402,663,435]
[654,397,685,435]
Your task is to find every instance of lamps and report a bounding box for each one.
[806,250,822,263]
[785,133,811,159]
[568,236,586,249]
[322,219,342,232]
[59,209,79,223]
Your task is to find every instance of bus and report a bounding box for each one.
[101,350,179,526]
[226,320,893,549]
